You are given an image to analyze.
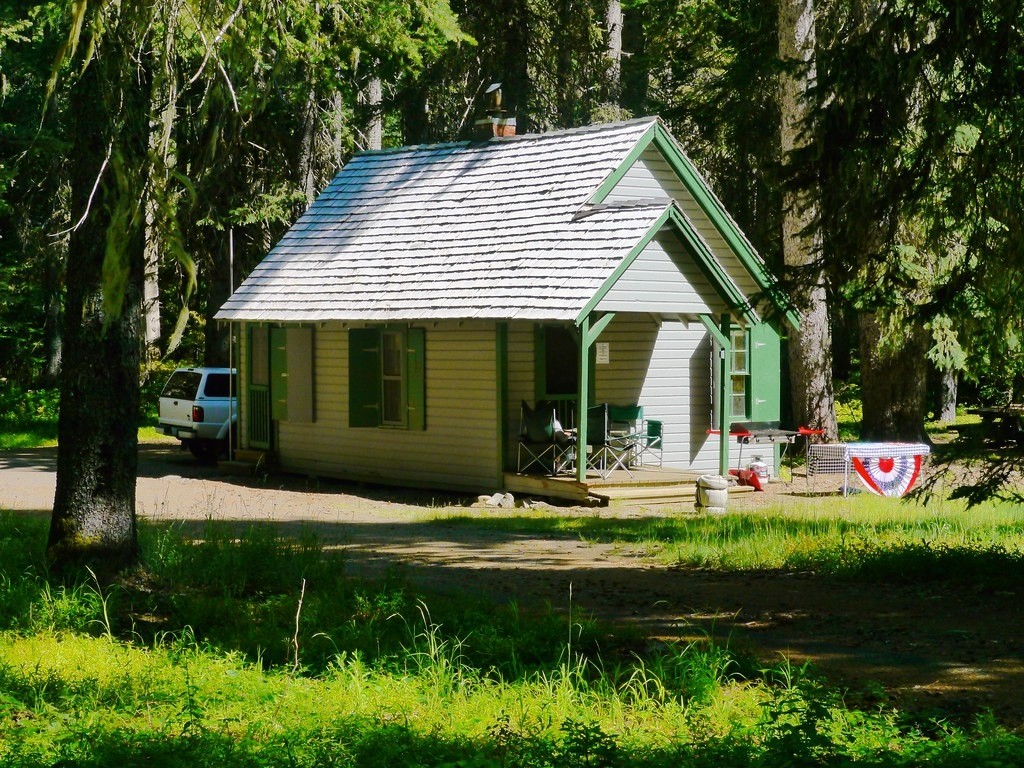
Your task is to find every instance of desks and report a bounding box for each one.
[810,441,930,499]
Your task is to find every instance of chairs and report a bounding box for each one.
[516,396,665,480]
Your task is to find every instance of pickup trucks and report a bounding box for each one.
[154,369,239,463]
